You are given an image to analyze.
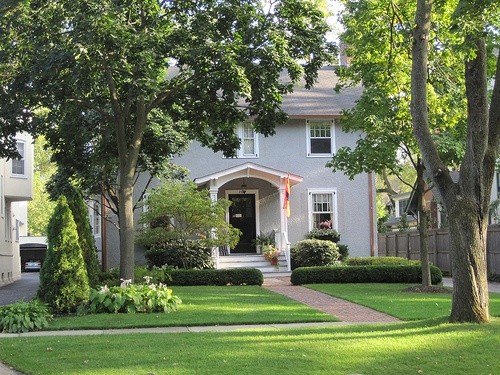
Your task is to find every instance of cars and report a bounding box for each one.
[24,259,41,272]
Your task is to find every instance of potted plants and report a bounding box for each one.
[251,230,281,270]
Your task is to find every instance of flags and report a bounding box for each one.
[282,176,291,218]
